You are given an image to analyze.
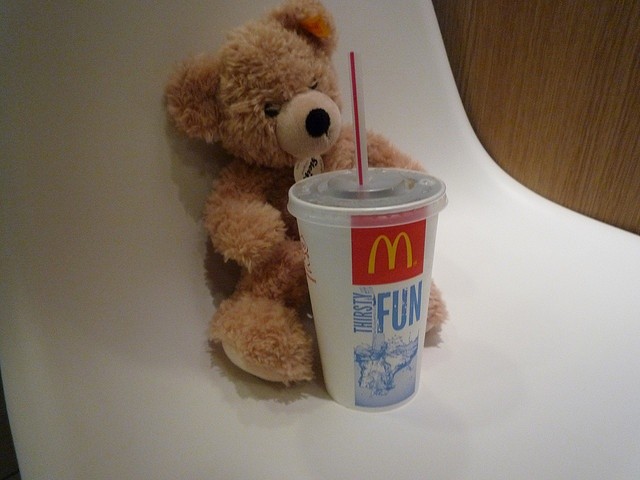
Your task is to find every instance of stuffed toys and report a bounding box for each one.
[164,0,448,385]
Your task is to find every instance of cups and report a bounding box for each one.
[286,166,449,415]
